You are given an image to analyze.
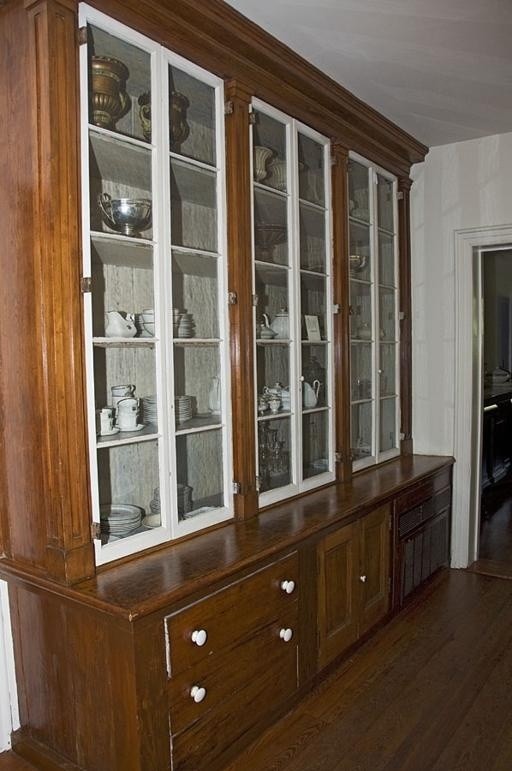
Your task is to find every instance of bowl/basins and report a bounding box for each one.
[142,307,182,335]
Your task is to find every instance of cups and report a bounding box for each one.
[96,384,140,433]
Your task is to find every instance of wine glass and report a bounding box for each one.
[257,399,281,415]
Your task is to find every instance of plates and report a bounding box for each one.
[98,428,119,437]
[135,315,152,337]
[174,314,195,337]
[143,395,192,431]
[100,504,142,535]
[120,424,144,431]
[142,513,183,530]
[183,506,218,519]
[149,485,193,514]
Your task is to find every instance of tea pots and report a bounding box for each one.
[262,308,289,339]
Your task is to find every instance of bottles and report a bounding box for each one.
[208,377,221,417]
